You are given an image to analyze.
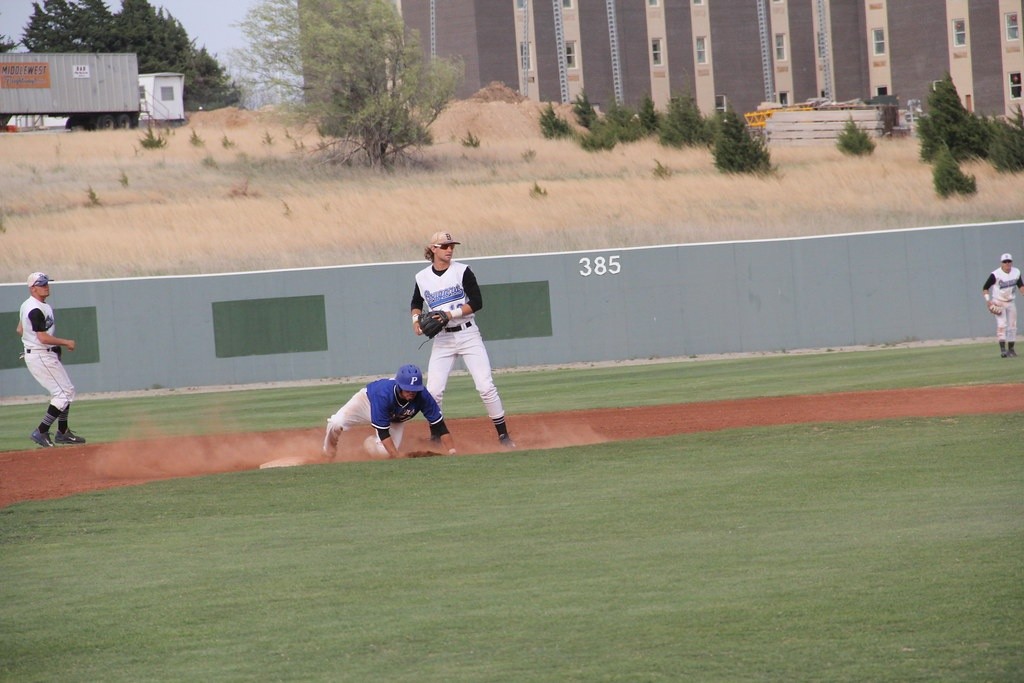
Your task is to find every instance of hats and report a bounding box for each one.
[1001,253,1013,261]
[430,231,461,245]
[27,272,56,288]
[396,364,425,392]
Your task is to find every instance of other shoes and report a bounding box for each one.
[1001,350,1018,358]
[501,436,516,449]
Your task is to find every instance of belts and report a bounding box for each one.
[445,321,473,332]
[27,348,55,353]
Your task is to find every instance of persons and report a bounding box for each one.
[323,364,456,459]
[16,272,85,447]
[411,232,517,448]
[983,253,1024,358]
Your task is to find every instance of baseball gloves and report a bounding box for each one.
[417,310,450,339]
[989,302,1004,316]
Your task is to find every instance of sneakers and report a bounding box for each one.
[54,428,87,443]
[30,428,55,448]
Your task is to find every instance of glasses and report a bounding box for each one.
[32,275,48,286]
[431,244,455,250]
[1002,260,1011,263]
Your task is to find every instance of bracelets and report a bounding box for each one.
[449,308,463,319]
[412,314,419,324]
[448,449,456,455]
